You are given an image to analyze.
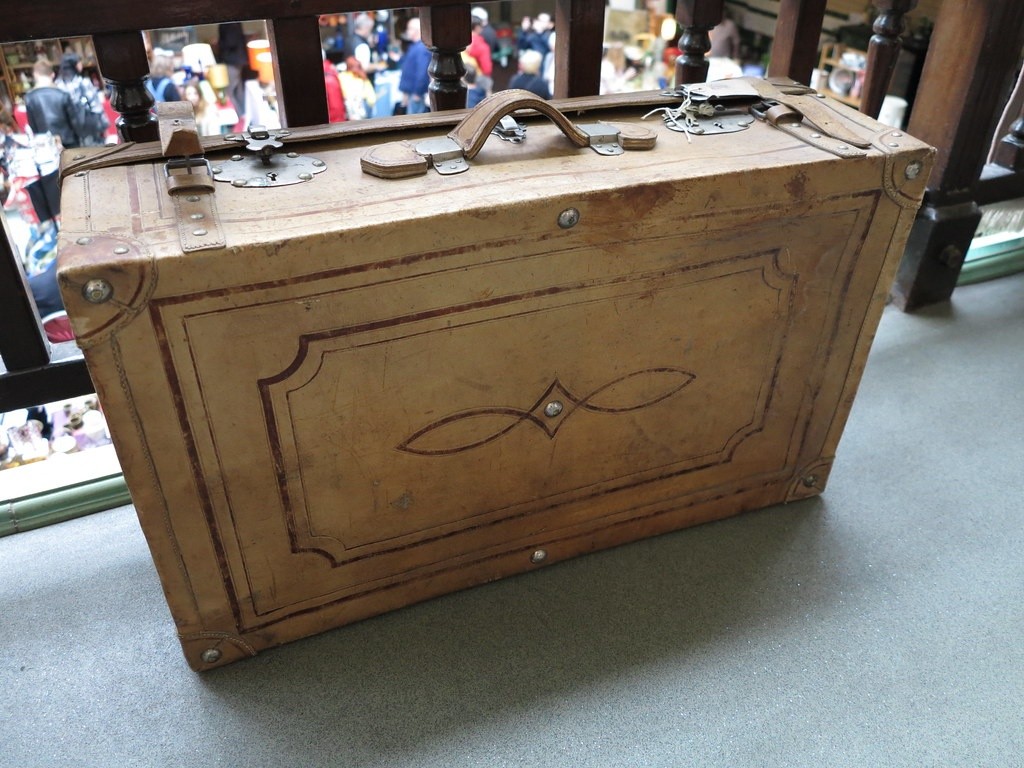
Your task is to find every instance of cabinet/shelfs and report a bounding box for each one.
[0,35,105,107]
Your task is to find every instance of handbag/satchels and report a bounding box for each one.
[82,102,102,130]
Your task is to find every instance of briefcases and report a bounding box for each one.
[57,75,936,670]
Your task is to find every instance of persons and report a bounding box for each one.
[24,6,743,151]
